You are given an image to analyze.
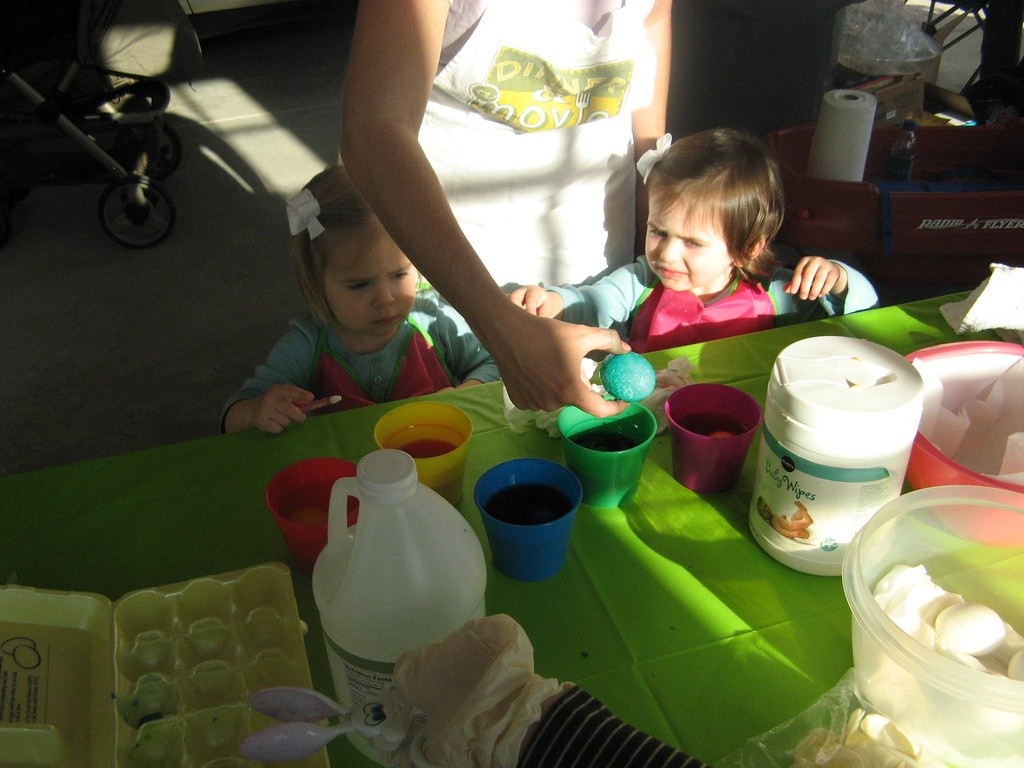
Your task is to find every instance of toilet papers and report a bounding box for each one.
[806,88,878,182]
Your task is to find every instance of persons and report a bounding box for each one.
[340,1,674,419]
[218,164,503,436]
[508,129,878,354]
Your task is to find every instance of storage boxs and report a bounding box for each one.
[1,562,330,768]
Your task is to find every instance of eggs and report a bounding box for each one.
[1007,648,1024,681]
[934,601,1005,657]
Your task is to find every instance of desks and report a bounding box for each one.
[1,287,1024,768]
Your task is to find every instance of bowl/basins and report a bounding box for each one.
[906,341,1024,548]
[842,484,1024,768]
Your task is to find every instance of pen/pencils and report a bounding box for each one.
[298,395,342,412]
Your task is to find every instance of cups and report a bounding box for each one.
[664,383,764,493]
[556,395,657,508]
[373,402,472,505]
[473,459,583,583]
[265,457,359,569]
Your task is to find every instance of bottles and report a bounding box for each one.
[312,448,487,766]
[887,120,917,182]
